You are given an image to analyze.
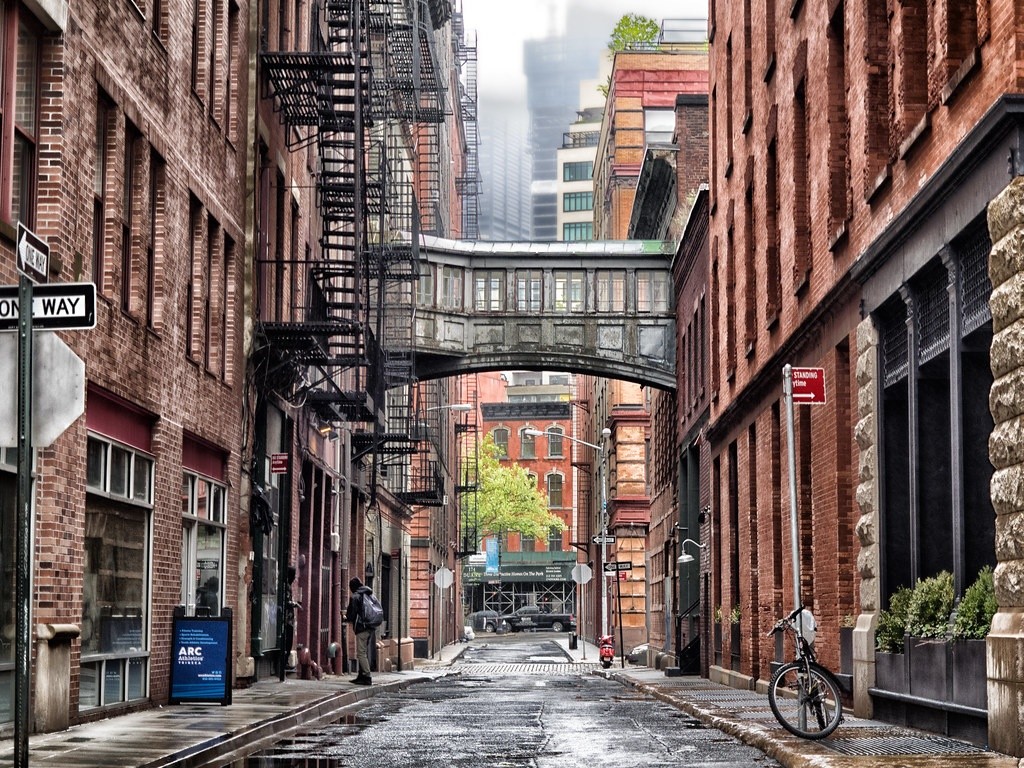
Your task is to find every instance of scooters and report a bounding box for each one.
[599,635,616,669]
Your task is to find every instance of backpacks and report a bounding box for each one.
[360,592,382,626]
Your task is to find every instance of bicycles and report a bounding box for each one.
[765,603,844,741]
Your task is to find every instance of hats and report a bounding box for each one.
[350,577,363,593]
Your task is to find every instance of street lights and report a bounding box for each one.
[526,425,612,637]
[404,402,472,639]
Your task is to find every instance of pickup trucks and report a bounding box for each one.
[485,606,573,632]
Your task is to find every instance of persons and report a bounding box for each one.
[343,578,372,685]
[282,567,303,670]
[196,576,219,614]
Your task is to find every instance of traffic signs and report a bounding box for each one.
[0,283,96,333]
[592,535,616,545]
[604,561,632,571]
[16,221,50,284]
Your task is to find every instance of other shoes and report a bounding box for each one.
[349,674,372,686]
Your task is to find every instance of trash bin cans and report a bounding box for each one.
[568,632,578,649]
